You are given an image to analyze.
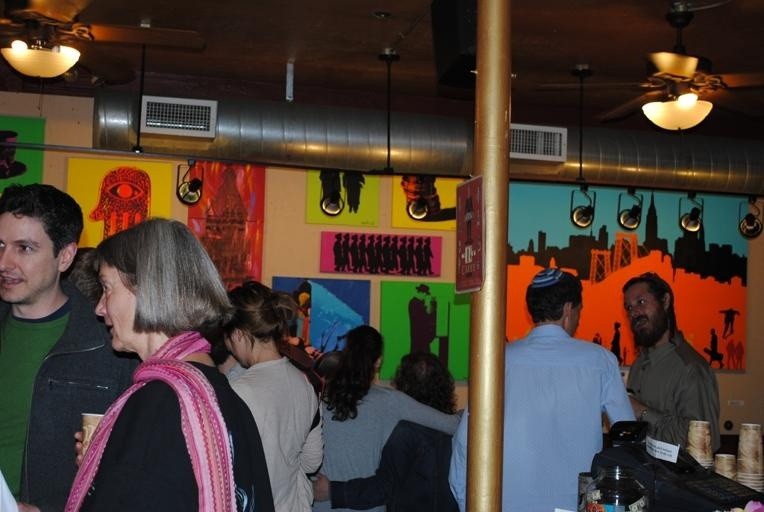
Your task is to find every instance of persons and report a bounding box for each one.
[620,272,721,460]
[310,325,463,512]
[63,216,276,511]
[446,268,636,512]
[0,182,143,512]
[0,131,27,179]
[218,281,324,512]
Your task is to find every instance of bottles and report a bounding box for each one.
[586,464,648,512]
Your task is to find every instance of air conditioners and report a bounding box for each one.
[138,91,219,142]
[507,121,569,165]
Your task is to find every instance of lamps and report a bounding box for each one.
[407,176,434,223]
[173,159,203,204]
[640,1,714,133]
[0,0,100,80]
[317,171,345,217]
[569,181,764,241]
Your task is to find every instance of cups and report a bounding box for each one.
[81,412,104,456]
[686,419,764,492]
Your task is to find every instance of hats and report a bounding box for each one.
[416,284,429,294]
[530,268,566,289]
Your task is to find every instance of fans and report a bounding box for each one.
[536,1,764,120]
[0,0,206,49]
